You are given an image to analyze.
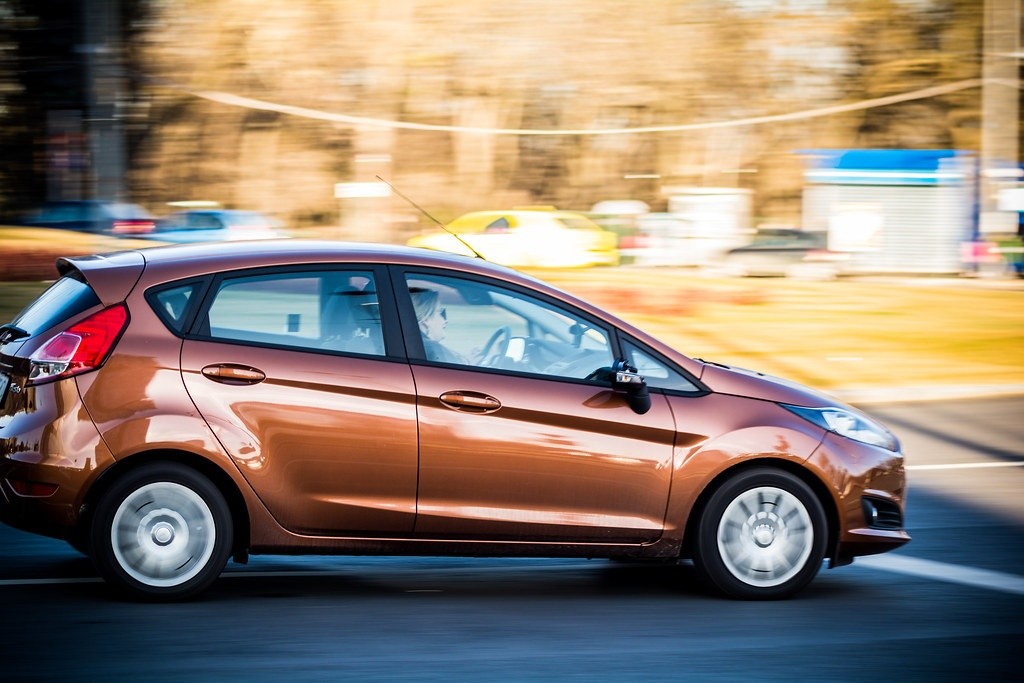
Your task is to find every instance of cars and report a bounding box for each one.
[407,207,620,269]
[145,210,284,244]
[0,244,911,600]
[8,199,160,239]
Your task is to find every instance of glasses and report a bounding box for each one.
[432,308,447,319]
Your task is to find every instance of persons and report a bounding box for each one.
[410,287,485,366]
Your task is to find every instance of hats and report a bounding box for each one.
[407,287,439,323]
